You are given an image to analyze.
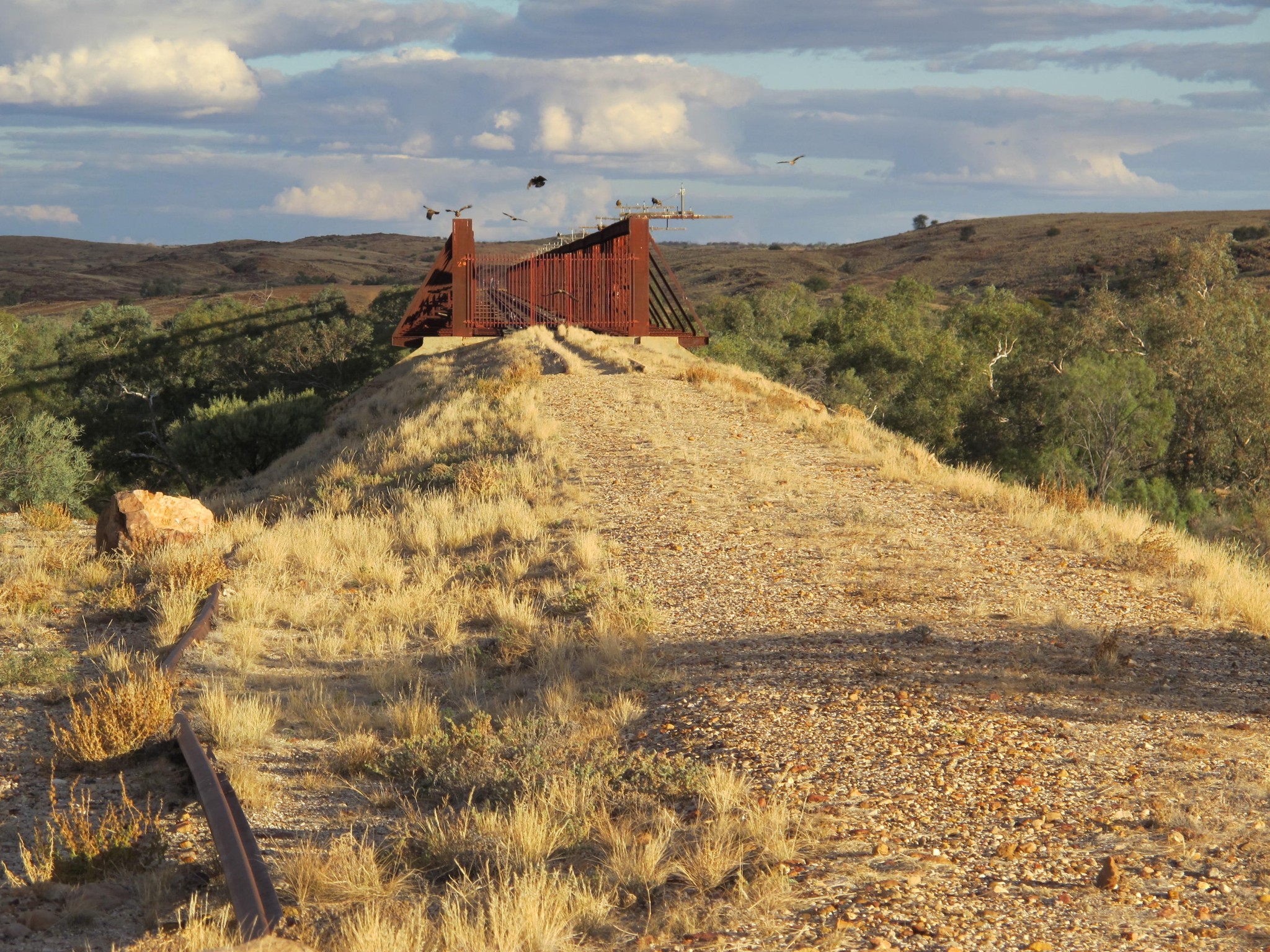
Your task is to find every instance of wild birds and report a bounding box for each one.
[527,175,548,190]
[652,198,659,205]
[777,155,804,165]
[424,206,440,220]
[596,223,607,231]
[616,199,621,206]
[446,203,473,218]
[503,213,528,223]
